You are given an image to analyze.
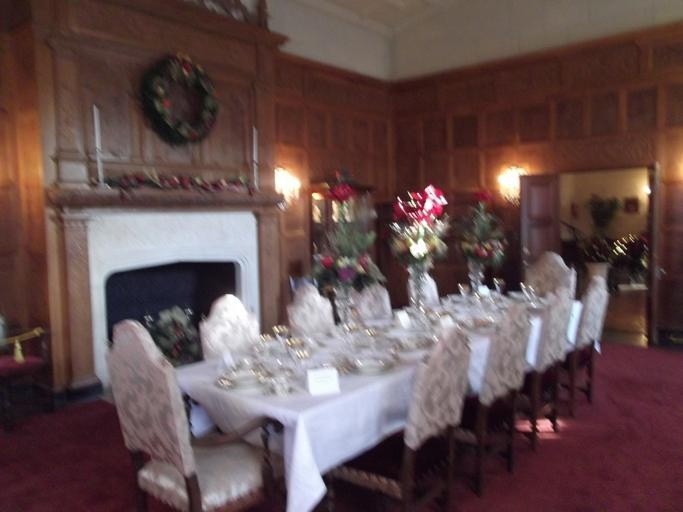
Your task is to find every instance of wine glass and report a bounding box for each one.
[213,268,543,399]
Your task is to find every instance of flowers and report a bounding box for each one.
[454,188,508,267]
[314,168,386,282]
[387,183,450,272]
